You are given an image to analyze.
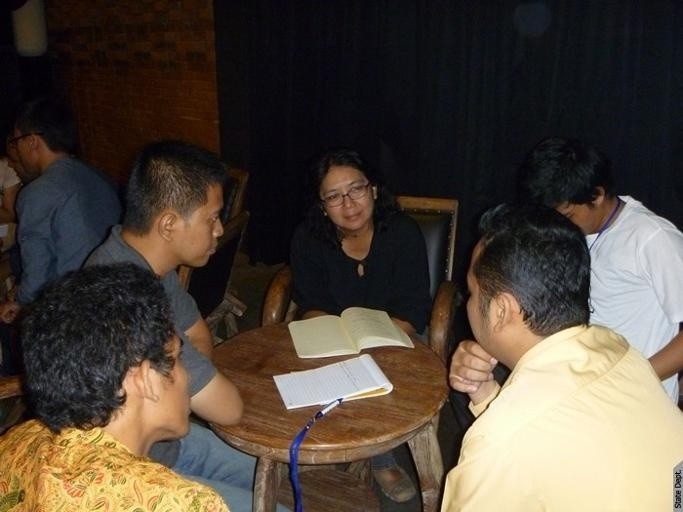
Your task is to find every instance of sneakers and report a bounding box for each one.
[371,466,418,502]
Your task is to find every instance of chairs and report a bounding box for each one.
[261,195,464,433]
[0,371,29,438]
[178,210,252,349]
[218,167,250,229]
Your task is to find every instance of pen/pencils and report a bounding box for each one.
[315,398,344,417]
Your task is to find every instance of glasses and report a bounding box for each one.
[5,131,43,149]
[317,183,371,207]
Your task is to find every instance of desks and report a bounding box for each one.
[205,320,452,512]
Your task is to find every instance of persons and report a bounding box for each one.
[294,145,432,505]
[510,133,682,405]
[439,199,683,512]
[75,139,285,510]
[1,92,123,377]
[0,261,235,512]
[0,155,23,280]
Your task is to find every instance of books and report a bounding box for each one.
[288,306,415,359]
[273,354,393,408]
